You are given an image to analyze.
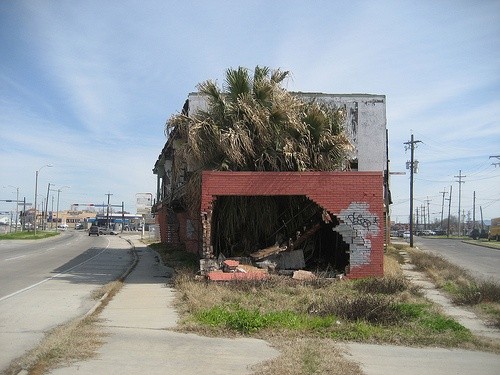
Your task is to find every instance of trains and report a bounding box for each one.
[45,216,89,229]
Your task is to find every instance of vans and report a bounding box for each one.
[88,218,117,236]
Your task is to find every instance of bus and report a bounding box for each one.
[416,229,437,236]
[97,213,144,232]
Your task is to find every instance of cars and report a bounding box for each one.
[0,217,14,224]
[12,222,38,230]
[390,229,410,238]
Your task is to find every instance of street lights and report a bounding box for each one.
[106,193,113,227]
[34,164,53,236]
